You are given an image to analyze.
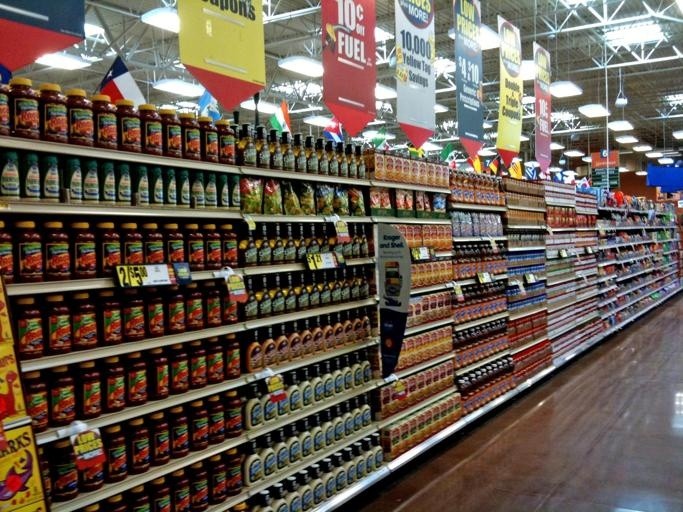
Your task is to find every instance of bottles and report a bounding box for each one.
[38,83,69,143]
[381,361,454,416]
[11,78,40,139]
[14,296,43,358]
[116,164,132,202]
[152,477,172,512]
[244,223,369,267]
[207,223,221,267]
[222,225,239,269]
[91,95,118,149]
[159,109,181,157]
[207,395,226,443]
[405,292,453,327]
[219,175,229,207]
[145,288,164,338]
[245,393,370,487]
[77,362,103,420]
[226,333,241,380]
[197,117,219,161]
[230,174,242,206]
[188,399,209,451]
[43,221,70,281]
[225,391,242,437]
[223,288,238,325]
[151,167,163,203]
[253,433,383,512]
[36,447,51,506]
[393,326,453,370]
[116,100,140,152]
[150,411,170,466]
[23,372,49,432]
[450,169,516,416]
[109,495,128,512]
[52,440,79,501]
[123,290,145,339]
[409,261,452,288]
[190,462,209,512]
[41,157,60,199]
[245,349,371,429]
[362,149,450,189]
[72,294,97,350]
[84,504,100,512]
[215,121,236,164]
[67,159,83,199]
[170,407,188,457]
[170,344,190,394]
[383,392,462,461]
[206,281,224,328]
[21,155,41,198]
[135,166,150,206]
[176,112,201,160]
[147,348,170,401]
[0,221,13,280]
[166,286,186,333]
[225,449,243,496]
[207,336,225,382]
[1,151,19,200]
[171,471,190,512]
[164,224,184,263]
[138,104,164,155]
[103,356,128,411]
[185,283,204,331]
[245,266,370,320]
[144,224,164,264]
[79,462,105,492]
[127,419,150,474]
[46,296,72,351]
[71,222,97,278]
[393,224,452,248]
[189,341,208,388]
[49,366,76,426]
[103,425,126,483]
[205,174,218,205]
[179,170,192,204]
[191,173,205,206]
[131,486,152,512]
[244,306,371,372]
[97,222,121,278]
[0,77,12,135]
[185,223,204,271]
[233,503,247,512]
[209,455,227,505]
[127,352,149,405]
[16,221,44,280]
[101,162,117,200]
[99,291,123,345]
[121,222,143,265]
[164,169,177,203]
[67,88,94,145]
[83,160,100,200]
[231,124,366,179]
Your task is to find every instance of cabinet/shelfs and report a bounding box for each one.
[598,185,682,336]
[374,153,607,473]
[0,73,391,512]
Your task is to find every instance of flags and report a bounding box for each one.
[508,160,522,180]
[268,100,293,139]
[489,155,510,177]
[98,55,146,112]
[440,143,458,164]
[373,126,389,152]
[538,166,591,189]
[198,91,224,127]
[467,154,481,175]
[406,142,425,159]
[526,167,538,181]
[323,116,345,147]
